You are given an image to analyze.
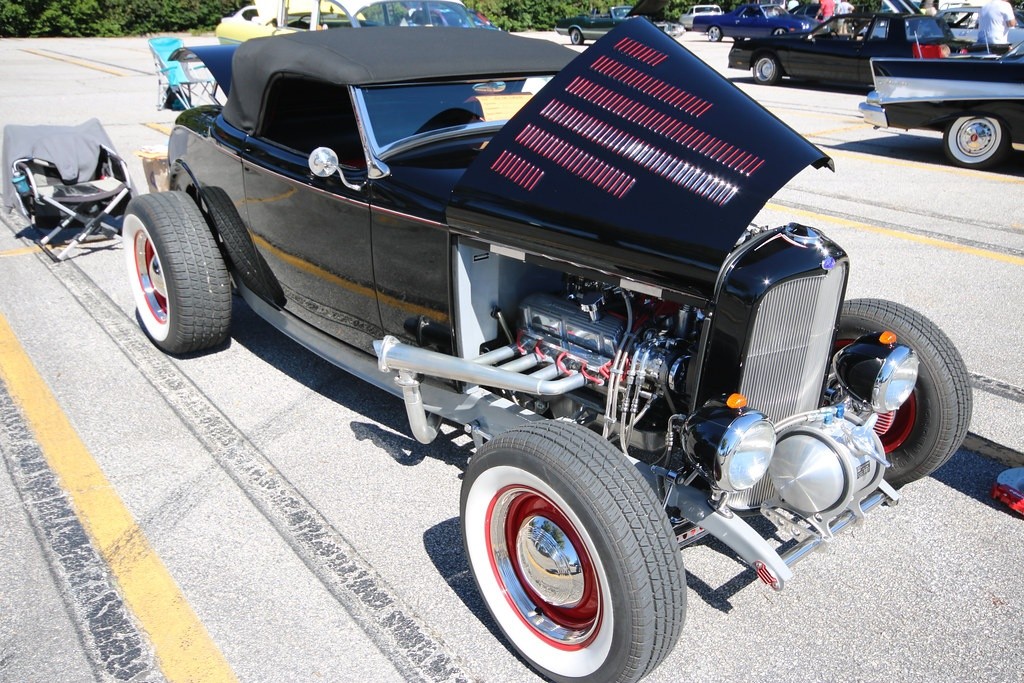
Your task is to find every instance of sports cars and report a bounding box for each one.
[554,0,687,46]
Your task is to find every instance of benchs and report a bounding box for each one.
[261,104,446,157]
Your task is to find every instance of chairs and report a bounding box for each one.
[2,117,137,263]
[148,37,223,111]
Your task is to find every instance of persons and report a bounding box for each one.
[976,0,1017,48]
[787,0,799,14]
[815,0,855,34]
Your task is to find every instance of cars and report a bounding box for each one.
[725,11,1015,92]
[691,4,829,44]
[214,0,546,99]
[882,0,1024,58]
[678,4,724,29]
[859,36,1024,174]
[118,13,974,683]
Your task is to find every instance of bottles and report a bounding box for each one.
[11,172,30,196]
